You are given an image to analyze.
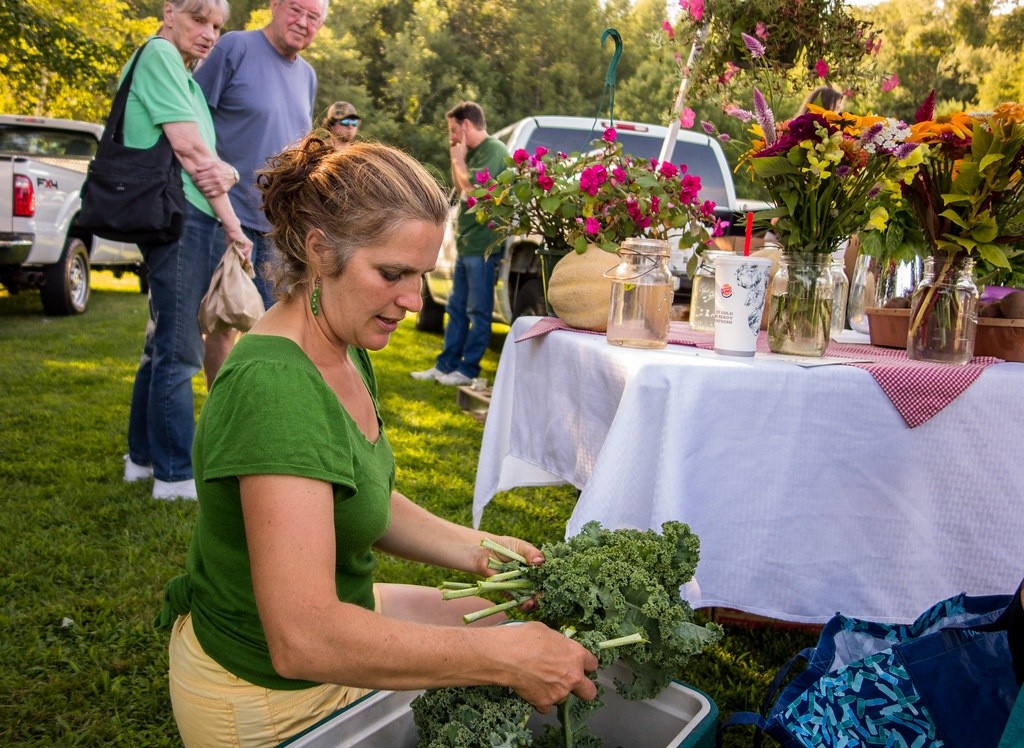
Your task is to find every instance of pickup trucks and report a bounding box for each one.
[0,114,151,317]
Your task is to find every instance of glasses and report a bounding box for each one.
[340,118,359,126]
[284,2,320,26]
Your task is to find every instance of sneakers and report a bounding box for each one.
[409,367,445,381]
[434,370,474,386]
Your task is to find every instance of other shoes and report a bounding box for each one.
[152,478,198,501]
[123,453,153,482]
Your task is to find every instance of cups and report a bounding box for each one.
[713,254,773,358]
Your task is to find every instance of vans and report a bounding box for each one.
[413,115,741,331]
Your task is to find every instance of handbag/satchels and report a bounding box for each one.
[720,577,1024,748]
[198,241,267,335]
[80,142,183,241]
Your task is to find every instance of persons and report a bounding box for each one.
[116,0,254,499]
[155,104,598,748]
[325,100,361,143]
[192,0,328,393]
[765,88,843,241]
[408,100,513,384]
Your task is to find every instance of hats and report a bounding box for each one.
[328,101,361,120]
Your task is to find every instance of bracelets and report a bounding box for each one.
[230,166,240,183]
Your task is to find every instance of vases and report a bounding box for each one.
[548,243,619,334]
[732,38,802,68]
[601,239,672,347]
[768,250,833,356]
[908,256,978,361]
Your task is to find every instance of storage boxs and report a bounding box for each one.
[277,663,723,748]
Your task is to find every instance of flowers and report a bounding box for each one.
[457,0,1024,352]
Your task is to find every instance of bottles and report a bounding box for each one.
[830,258,850,337]
[603,237,673,348]
[689,250,739,333]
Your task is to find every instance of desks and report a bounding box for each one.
[473,315,1024,623]
